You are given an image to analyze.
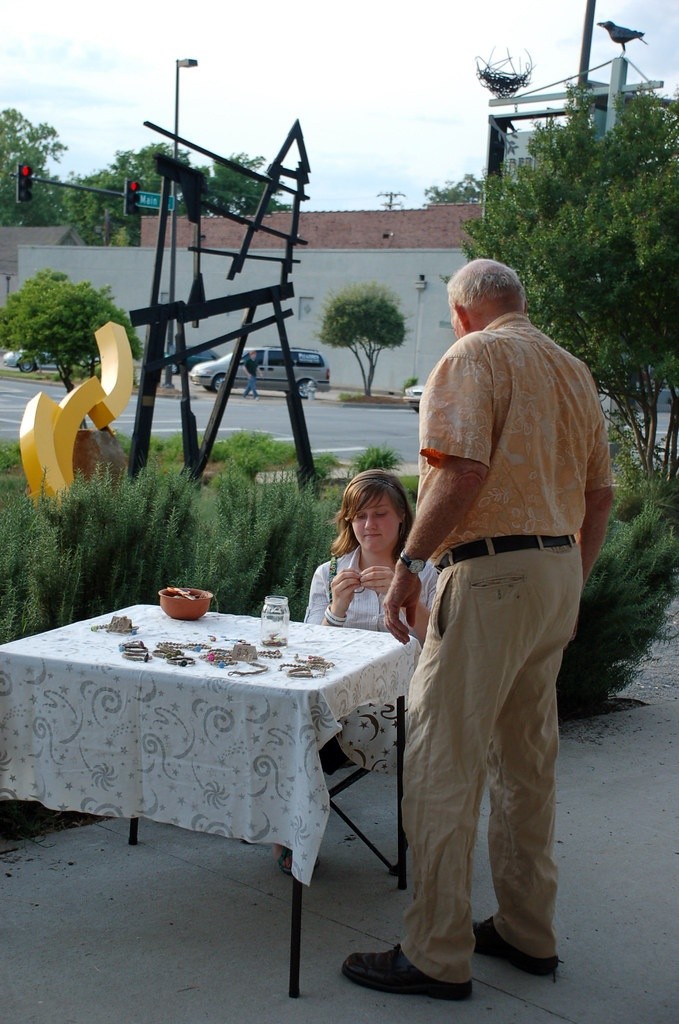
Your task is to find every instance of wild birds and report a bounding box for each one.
[598,20,648,57]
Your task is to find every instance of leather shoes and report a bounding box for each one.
[473,916,558,976]
[342,944,471,1001]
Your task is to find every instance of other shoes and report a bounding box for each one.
[254,396,259,401]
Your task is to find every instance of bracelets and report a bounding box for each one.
[325,605,347,627]
[352,574,365,593]
[92,623,334,679]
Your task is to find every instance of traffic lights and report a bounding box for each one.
[124,179,143,217]
[15,163,34,204]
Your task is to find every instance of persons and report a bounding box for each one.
[342,258,615,998]
[242,351,263,400]
[272,469,440,874]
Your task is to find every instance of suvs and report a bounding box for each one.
[189,346,333,399]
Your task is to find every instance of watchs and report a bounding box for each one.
[399,550,426,573]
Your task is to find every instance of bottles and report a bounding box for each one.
[285,596,290,651]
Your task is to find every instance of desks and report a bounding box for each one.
[0,604,422,998]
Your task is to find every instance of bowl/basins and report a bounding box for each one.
[158,587,213,620]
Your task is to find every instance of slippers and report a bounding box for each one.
[276,846,319,875]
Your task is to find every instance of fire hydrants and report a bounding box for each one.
[306,379,318,401]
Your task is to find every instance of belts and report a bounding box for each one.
[436,535,575,575]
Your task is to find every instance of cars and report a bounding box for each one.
[3,347,102,374]
[161,344,223,376]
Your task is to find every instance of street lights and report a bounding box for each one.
[161,58,200,390]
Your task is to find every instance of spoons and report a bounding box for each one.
[167,587,196,601]
